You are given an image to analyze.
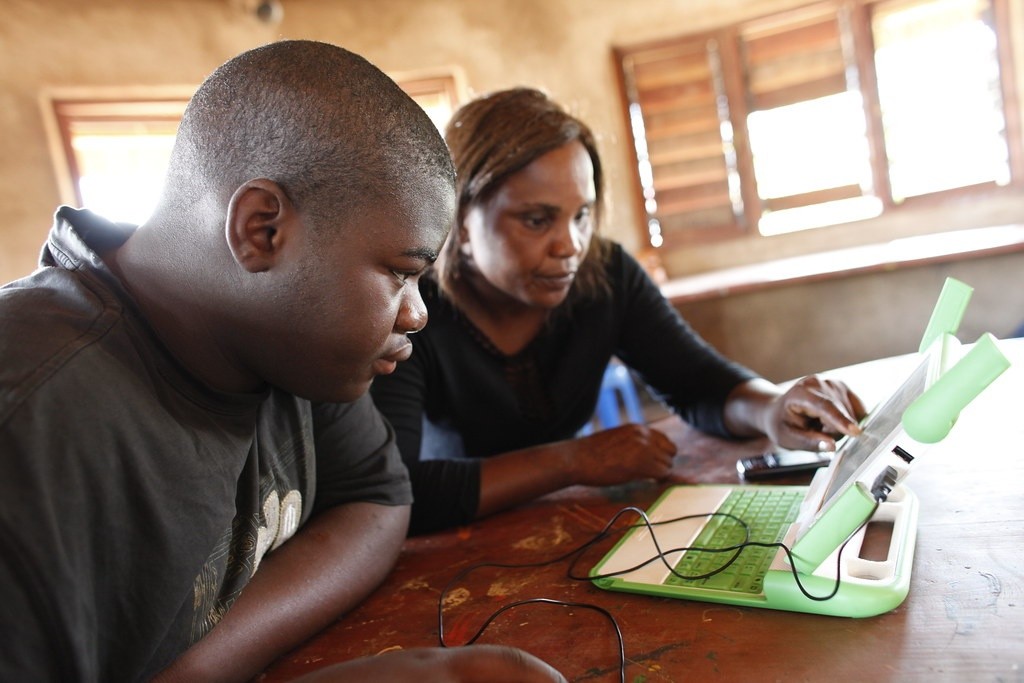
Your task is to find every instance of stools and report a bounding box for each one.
[575,366,645,438]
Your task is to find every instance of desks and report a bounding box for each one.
[659,223,1024,380]
[255,335,1024,683]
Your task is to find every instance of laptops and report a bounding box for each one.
[586,277,1012,619]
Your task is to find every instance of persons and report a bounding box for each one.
[369,86,866,544]
[0,41,455,683]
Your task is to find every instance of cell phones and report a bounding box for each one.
[737,452,833,481]
